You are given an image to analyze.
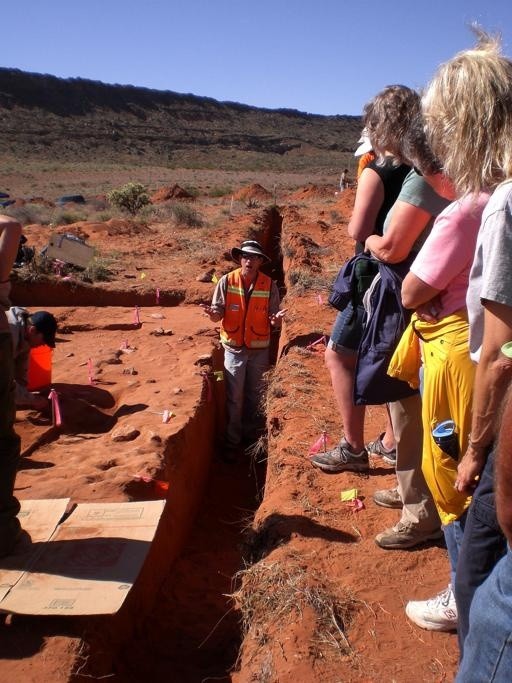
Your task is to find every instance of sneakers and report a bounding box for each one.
[373,485,403,508]
[406,584,459,631]
[374,522,444,550]
[365,431,396,465]
[309,437,368,472]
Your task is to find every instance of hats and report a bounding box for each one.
[231,240,272,265]
[32,311,57,348]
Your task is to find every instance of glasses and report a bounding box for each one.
[241,252,260,261]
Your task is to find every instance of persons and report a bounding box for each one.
[200,240,289,461]
[311,86,439,472]
[455,180,511,682]
[2,304,57,482]
[366,117,451,550]
[0,212,32,569]
[406,23,511,630]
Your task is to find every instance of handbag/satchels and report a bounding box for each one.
[353,260,378,301]
